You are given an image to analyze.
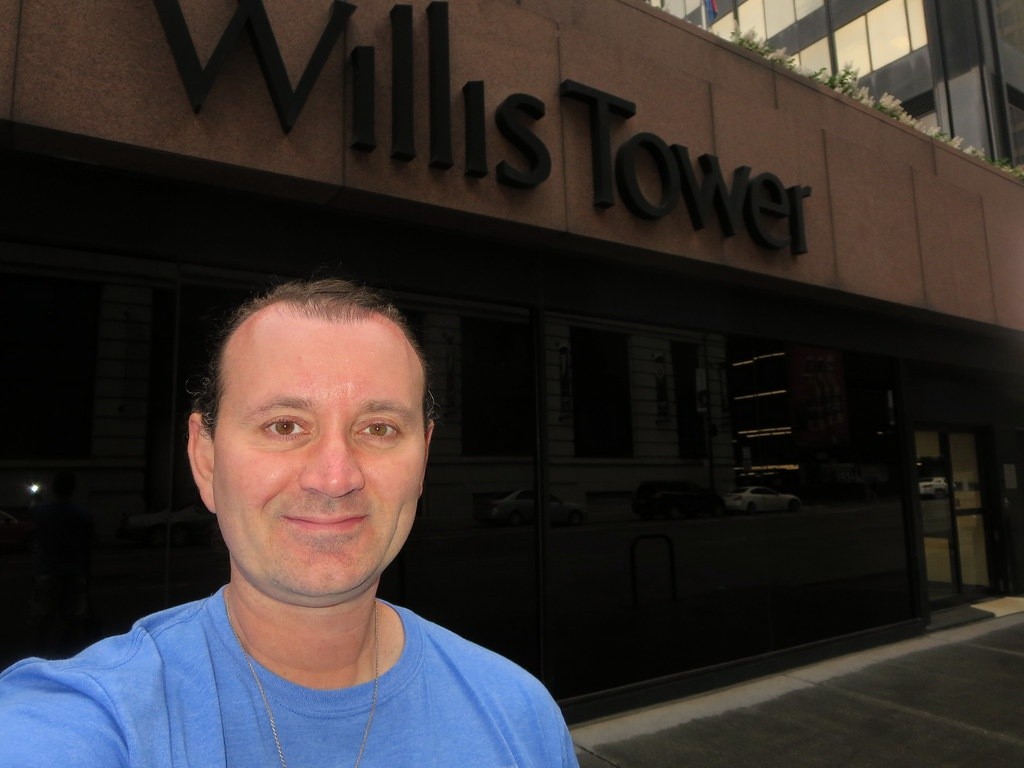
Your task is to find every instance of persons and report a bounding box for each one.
[0,279,579,768]
[29,471,94,654]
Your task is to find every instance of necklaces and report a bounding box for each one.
[226,602,378,768]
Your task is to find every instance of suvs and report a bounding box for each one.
[632,481,722,519]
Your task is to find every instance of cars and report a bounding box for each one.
[482,489,584,528]
[118,507,226,546]
[725,487,803,512]
[918,476,951,499]
